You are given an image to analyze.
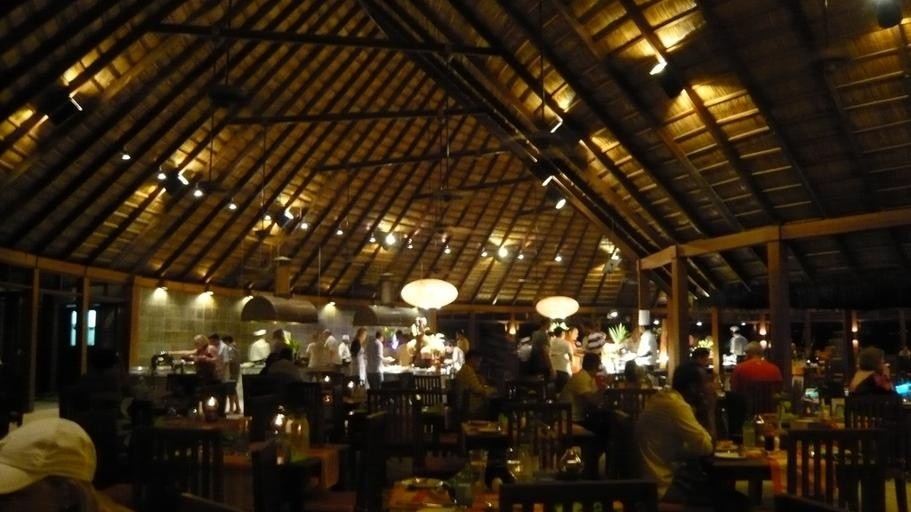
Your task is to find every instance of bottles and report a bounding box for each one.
[754,414,781,453]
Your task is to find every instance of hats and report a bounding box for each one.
[341,334,350,341]
[0,416,98,493]
[253,329,267,337]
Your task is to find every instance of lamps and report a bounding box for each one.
[87,310,97,346]
[45,0,703,336]
[71,310,77,346]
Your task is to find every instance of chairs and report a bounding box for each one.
[58,339,911,511]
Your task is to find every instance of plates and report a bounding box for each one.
[403,475,444,488]
[715,443,746,460]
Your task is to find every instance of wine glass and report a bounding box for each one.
[466,446,584,493]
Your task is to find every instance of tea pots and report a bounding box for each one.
[447,468,483,506]
[265,403,313,453]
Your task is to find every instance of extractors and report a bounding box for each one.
[352,270,427,328]
[243,254,320,324]
